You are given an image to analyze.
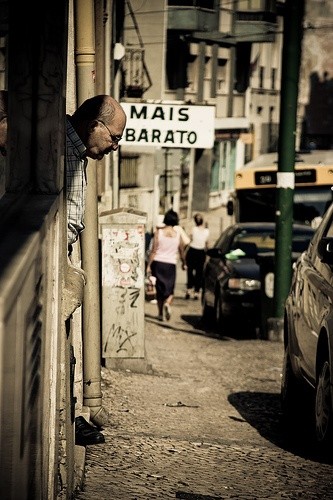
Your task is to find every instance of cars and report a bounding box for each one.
[201,222,318,324]
[280,204,332,440]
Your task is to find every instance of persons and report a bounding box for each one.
[0,95,127,446]
[146,208,186,322]
[185,213,210,301]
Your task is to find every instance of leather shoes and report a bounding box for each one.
[75,416,104,444]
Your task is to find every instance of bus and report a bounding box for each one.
[226,149,333,248]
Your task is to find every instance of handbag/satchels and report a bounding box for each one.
[144,278,157,301]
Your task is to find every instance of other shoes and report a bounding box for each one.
[158,315,163,321]
[194,296,198,300]
[184,294,191,300]
[164,303,171,320]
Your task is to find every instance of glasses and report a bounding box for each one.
[95,119,122,143]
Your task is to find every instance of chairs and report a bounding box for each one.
[235,242,258,256]
